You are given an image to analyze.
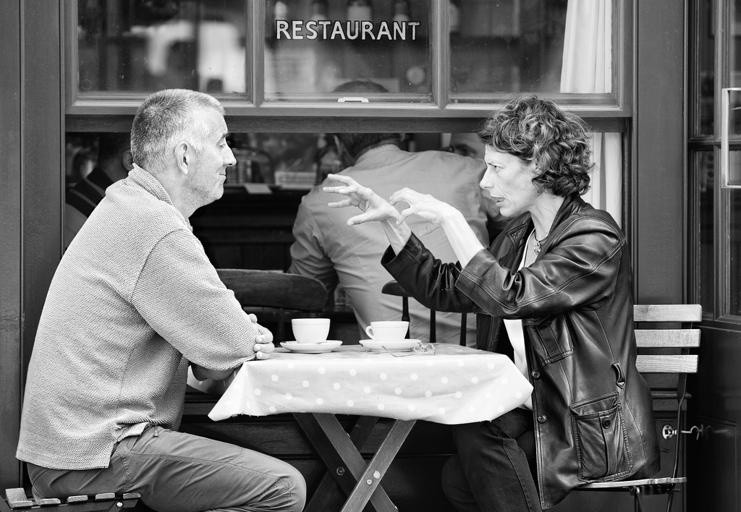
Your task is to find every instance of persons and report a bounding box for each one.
[62,110,139,250]
[288,79,496,346]
[13,85,311,512]
[320,92,667,511]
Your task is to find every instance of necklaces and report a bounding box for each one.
[532,229,550,255]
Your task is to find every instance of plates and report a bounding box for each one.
[279,339,343,354]
[359,338,421,353]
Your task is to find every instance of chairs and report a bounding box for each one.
[212,265,327,342]
[552,303,703,512]
[382,280,477,347]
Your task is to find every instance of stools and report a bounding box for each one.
[0,486,141,512]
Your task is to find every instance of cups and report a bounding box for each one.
[292,318,330,343]
[366,321,409,342]
